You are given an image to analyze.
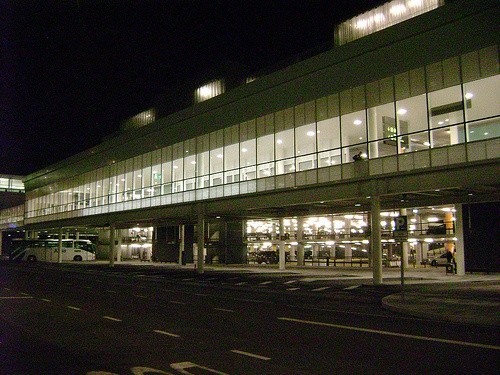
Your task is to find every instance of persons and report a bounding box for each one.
[444,247,453,263]
[352,151,363,161]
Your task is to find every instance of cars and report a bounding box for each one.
[431,252,447,267]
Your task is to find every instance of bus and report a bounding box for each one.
[10,238,96,263]
